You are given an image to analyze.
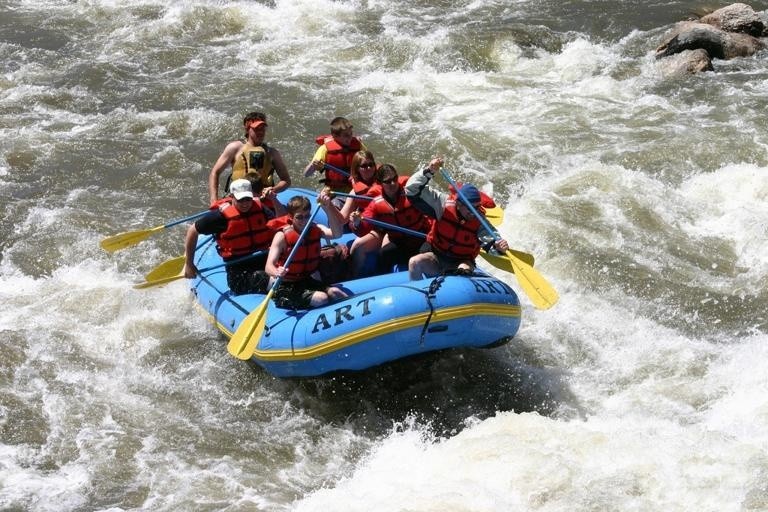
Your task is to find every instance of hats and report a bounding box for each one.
[245,120,268,129]
[457,184,481,203]
[230,179,253,201]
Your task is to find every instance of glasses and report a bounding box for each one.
[360,162,375,168]
[382,175,398,184]
[296,214,310,220]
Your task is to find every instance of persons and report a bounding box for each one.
[403,158,510,281]
[208,111,292,209]
[304,116,369,226]
[316,150,401,274]
[355,164,433,276]
[183,178,277,294]
[263,194,350,309]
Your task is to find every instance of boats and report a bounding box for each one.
[186,187,521,382]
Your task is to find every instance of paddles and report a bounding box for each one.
[357,217,533,274]
[142,235,212,282]
[226,202,322,361]
[328,188,505,228]
[438,167,559,310]
[103,208,209,253]
[132,251,269,291]
